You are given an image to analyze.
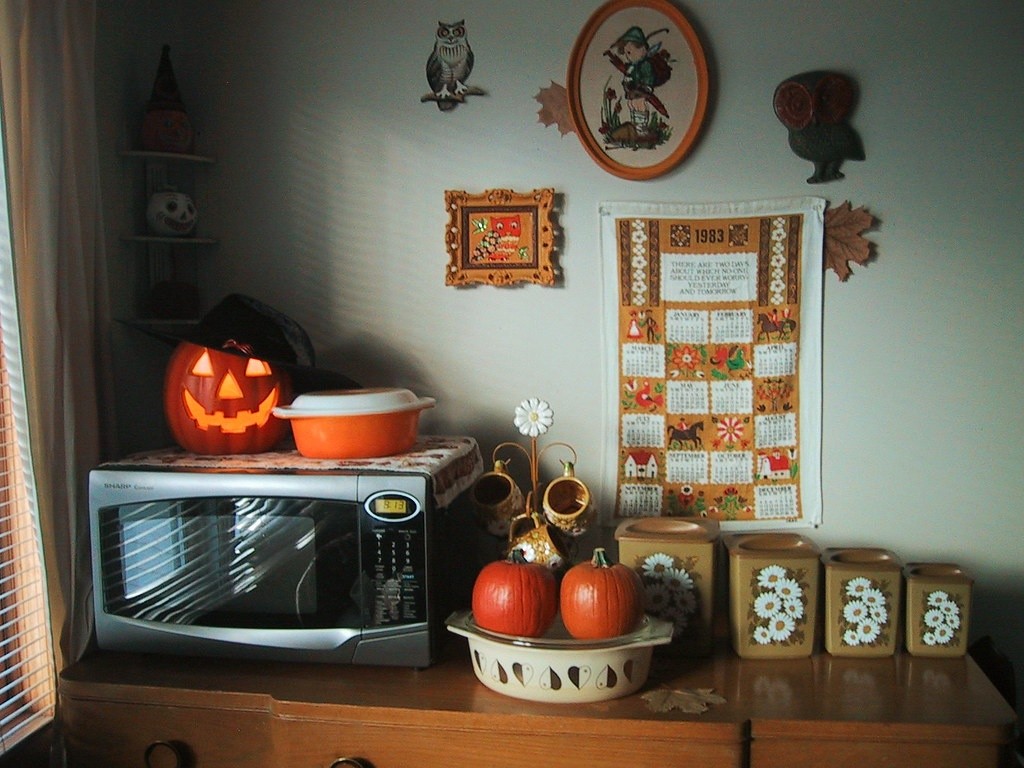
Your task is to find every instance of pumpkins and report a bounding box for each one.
[560,548,645,640]
[472,548,560,638]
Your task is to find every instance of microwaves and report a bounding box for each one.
[87,436,484,670]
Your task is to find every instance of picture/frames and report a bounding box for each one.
[443,187,555,288]
[566,0,708,182]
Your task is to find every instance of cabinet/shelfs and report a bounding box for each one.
[56,636,1024,768]
[114,111,218,340]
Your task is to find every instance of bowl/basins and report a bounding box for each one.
[270,388,437,459]
[441,610,675,705]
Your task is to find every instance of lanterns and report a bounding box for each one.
[166,343,295,451]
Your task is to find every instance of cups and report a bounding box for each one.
[613,514,974,660]
[469,459,526,541]
[497,512,575,580]
[542,462,600,541]
